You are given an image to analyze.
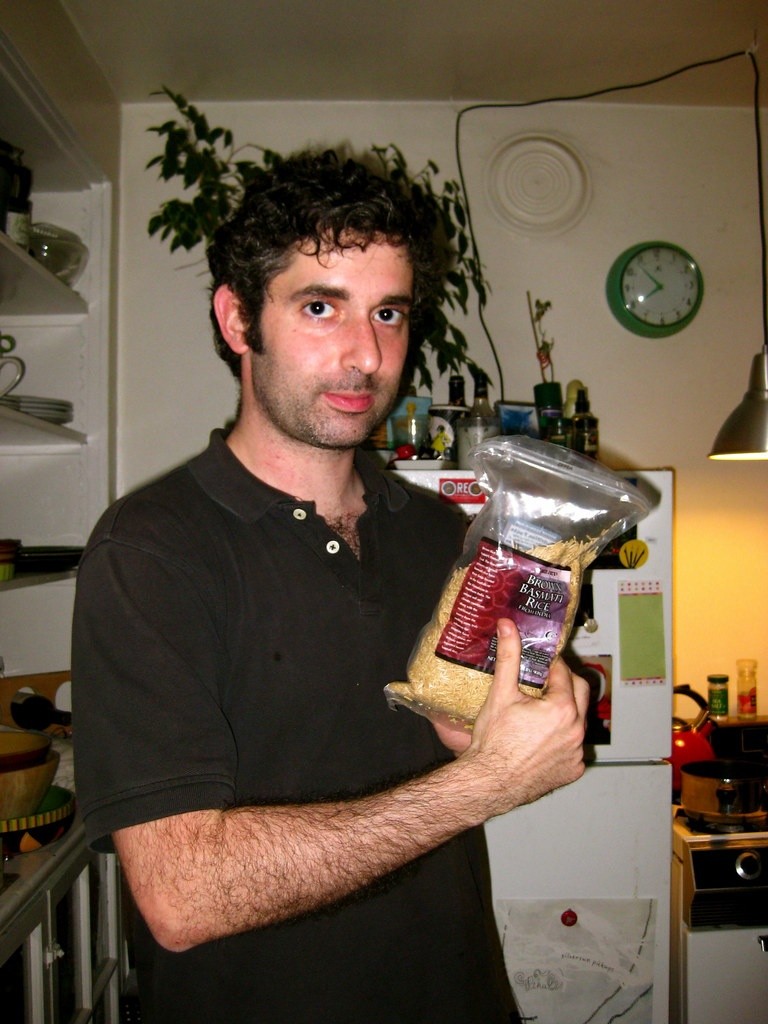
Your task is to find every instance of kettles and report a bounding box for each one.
[672,684,716,805]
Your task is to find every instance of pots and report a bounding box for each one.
[678,760,768,826]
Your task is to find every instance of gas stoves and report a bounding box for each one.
[671,715,768,862]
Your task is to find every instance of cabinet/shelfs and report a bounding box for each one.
[0,63,122,601]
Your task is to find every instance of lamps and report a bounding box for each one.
[708,53,768,462]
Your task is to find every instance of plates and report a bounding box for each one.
[13,546,86,575]
[0,394,74,426]
[394,461,455,472]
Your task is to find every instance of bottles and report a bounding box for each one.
[707,674,729,721]
[469,373,494,417]
[548,419,570,448]
[446,375,468,407]
[570,391,598,460]
[10,691,72,729]
[735,660,758,720]
[0,151,32,251]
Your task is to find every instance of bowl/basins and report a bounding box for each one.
[0,749,60,820]
[27,238,89,288]
[0,733,52,774]
[0,786,78,856]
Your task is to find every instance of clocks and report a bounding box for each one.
[600,240,706,339]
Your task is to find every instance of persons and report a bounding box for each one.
[69,141,590,1024]
[431,425,450,458]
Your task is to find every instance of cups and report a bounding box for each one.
[428,406,472,463]
[392,415,429,457]
[456,417,500,469]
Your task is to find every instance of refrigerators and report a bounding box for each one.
[390,469,677,1023]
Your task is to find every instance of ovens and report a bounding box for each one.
[670,833,768,1024]
